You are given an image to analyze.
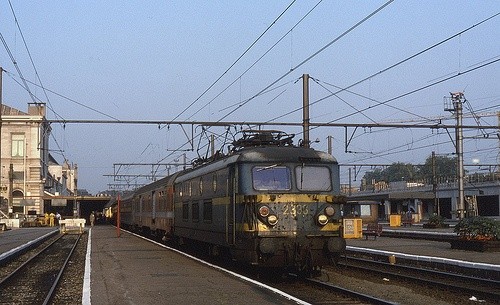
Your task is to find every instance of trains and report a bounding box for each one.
[104,128,347,278]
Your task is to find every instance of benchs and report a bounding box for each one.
[363,224,383,240]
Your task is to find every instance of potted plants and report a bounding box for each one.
[451,216,500,252]
[423,216,442,228]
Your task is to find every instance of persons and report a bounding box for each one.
[45,211,62,227]
[89,212,95,226]
